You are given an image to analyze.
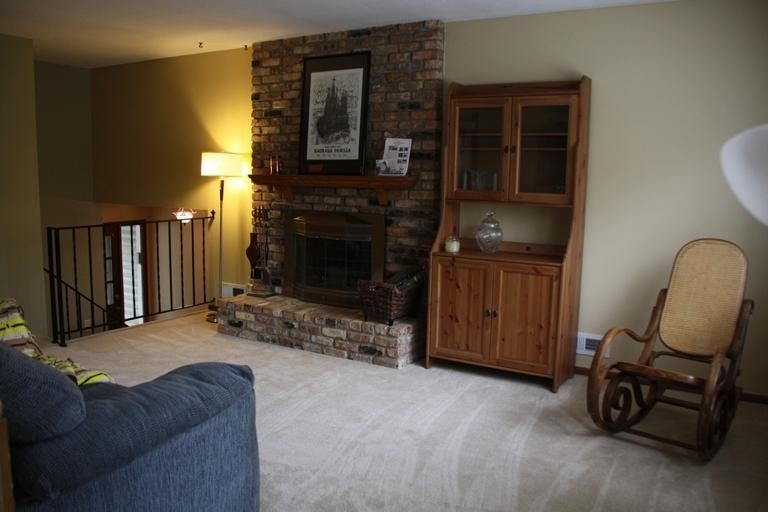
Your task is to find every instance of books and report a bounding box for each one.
[460,168,499,190]
[376,137,414,178]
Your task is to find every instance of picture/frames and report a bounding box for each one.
[298,48,371,175]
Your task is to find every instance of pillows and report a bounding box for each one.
[0,297,51,365]
[0,339,87,444]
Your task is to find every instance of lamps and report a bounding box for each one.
[172,207,198,227]
[200,151,249,311]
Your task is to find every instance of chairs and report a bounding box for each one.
[586,238,754,462]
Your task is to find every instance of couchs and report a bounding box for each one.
[0,361,261,512]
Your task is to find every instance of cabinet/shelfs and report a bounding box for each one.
[425,72,592,392]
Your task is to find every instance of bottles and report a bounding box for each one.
[444,233,460,253]
[475,209,502,255]
[268,154,280,175]
